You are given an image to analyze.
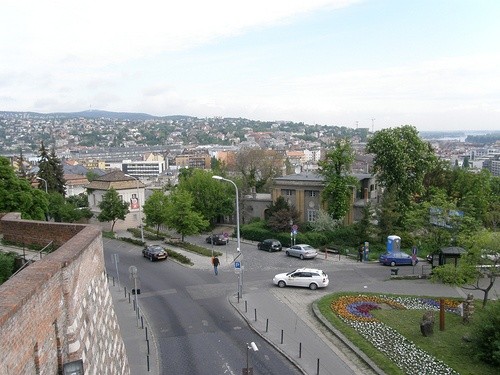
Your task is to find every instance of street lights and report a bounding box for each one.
[125,174,144,243]
[212,175,241,253]
[35,176,48,193]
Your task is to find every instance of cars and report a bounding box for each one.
[379,251,418,266]
[273,267,329,290]
[258,239,282,252]
[285,244,317,260]
[142,244,168,262]
[206,234,230,246]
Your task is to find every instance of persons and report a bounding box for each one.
[212,255,220,276]
[359,244,364,262]
